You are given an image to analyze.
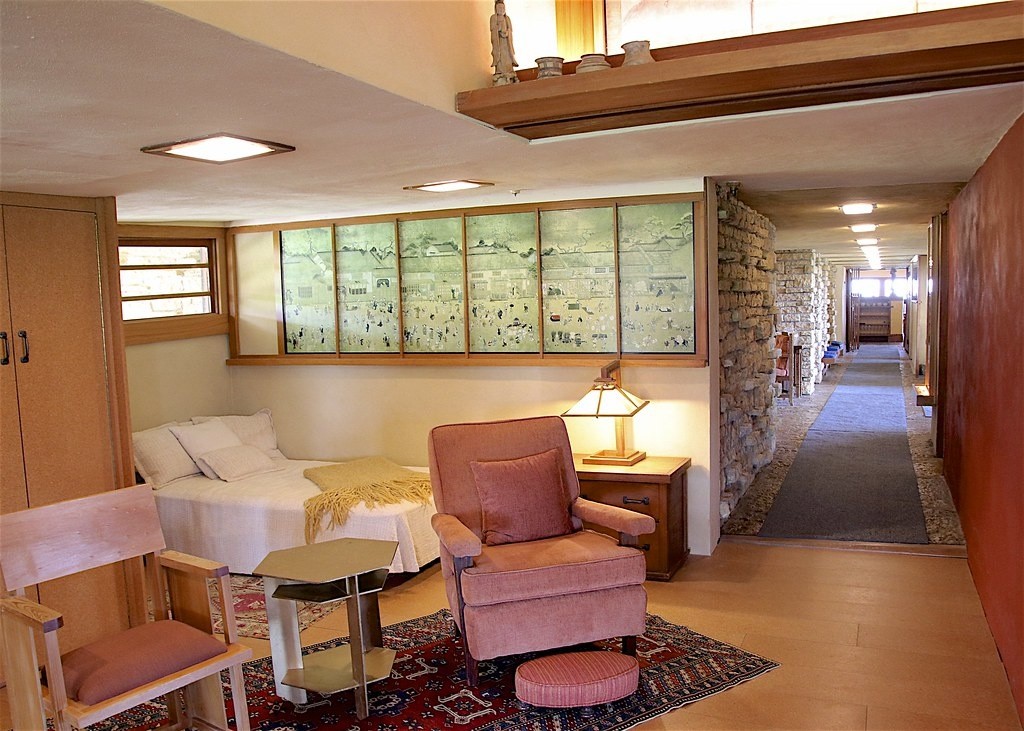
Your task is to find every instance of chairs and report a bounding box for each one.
[429,415,656,687]
[0,483,254,731]
[773,334,793,407]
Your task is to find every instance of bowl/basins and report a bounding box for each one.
[860,302,888,307]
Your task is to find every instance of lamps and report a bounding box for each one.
[560,360,651,468]
[840,202,881,245]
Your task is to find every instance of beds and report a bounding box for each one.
[130,407,449,592]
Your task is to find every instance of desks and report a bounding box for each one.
[252,537,401,720]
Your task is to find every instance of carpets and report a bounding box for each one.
[755,346,932,546]
[45,606,781,731]
[145,572,346,641]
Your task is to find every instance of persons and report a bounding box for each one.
[490,0,518,74]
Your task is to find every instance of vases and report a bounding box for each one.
[535,40,656,79]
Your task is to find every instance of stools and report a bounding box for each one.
[515,650,639,719]
[821,341,843,364]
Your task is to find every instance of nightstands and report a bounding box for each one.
[572,452,692,582]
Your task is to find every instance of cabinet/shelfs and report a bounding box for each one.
[0,190,150,689]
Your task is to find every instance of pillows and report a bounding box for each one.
[467,446,586,545]
[132,420,203,490]
[199,444,285,484]
[169,417,244,481]
[190,408,288,460]
